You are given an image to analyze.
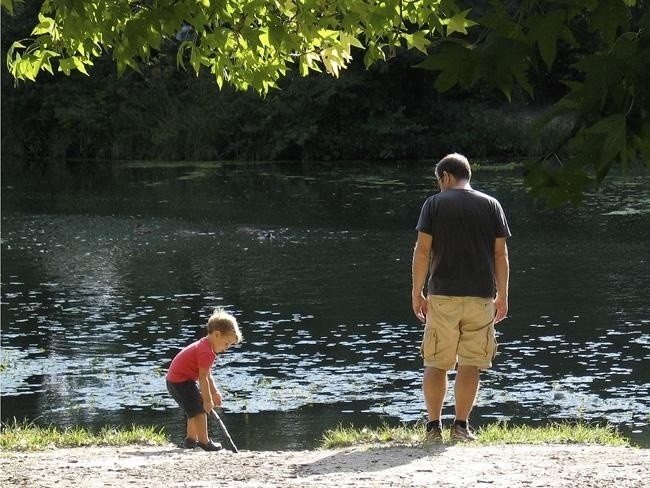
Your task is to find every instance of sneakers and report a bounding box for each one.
[425,421,445,445]
[195,437,222,451]
[183,437,196,448]
[450,418,478,443]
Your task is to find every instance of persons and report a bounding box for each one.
[166,308,241,451]
[411,153,512,441]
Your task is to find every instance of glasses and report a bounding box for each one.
[434,174,445,185]
